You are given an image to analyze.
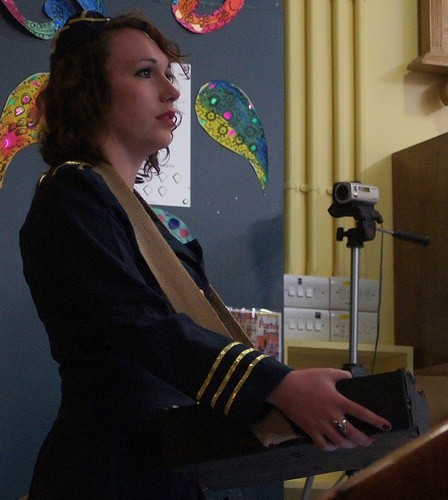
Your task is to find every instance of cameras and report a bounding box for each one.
[334,182,379,204]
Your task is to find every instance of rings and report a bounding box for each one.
[334,420,348,433]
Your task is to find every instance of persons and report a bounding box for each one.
[19,11,391,500]
